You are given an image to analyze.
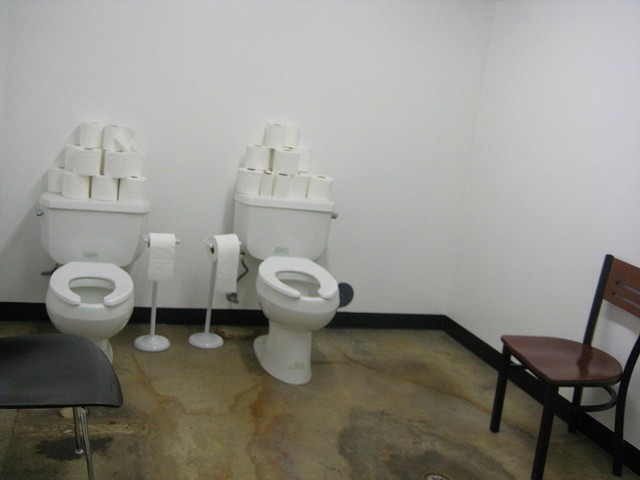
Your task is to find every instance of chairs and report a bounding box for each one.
[490,254,638,479]
[0,334,122,479]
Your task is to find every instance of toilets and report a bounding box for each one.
[38,191,149,365]
[233,191,340,386]
